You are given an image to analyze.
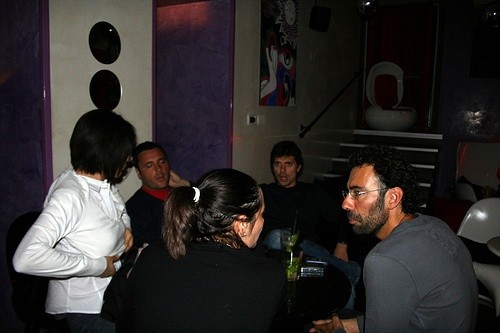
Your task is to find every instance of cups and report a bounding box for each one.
[281,227,300,252]
[284,256,302,282]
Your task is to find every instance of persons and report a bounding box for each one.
[11,109,134,333]
[123,140,198,247]
[114,167,351,333]
[309,142,481,333]
[257,139,353,264]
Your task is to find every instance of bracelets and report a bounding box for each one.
[338,240,349,245]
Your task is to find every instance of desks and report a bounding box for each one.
[270,249,353,333]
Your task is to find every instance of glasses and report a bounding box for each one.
[342,188,389,200]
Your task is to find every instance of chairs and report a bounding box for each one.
[6,211,72,333]
[364,60,418,130]
